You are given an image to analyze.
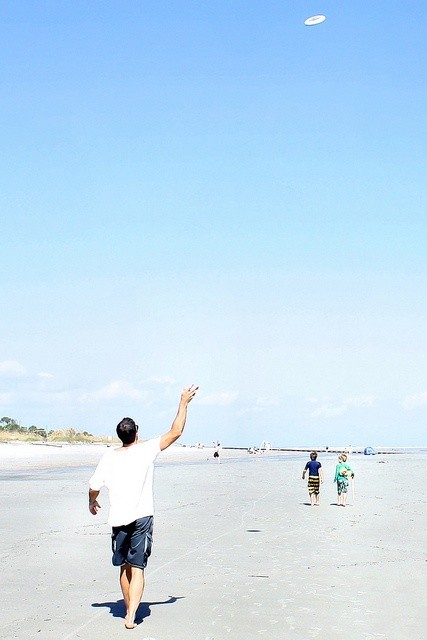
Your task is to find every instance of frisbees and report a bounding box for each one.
[303,14,328,27]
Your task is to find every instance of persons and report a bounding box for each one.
[207,441,222,464]
[302,453,323,506]
[88,383,199,629]
[334,454,354,507]
